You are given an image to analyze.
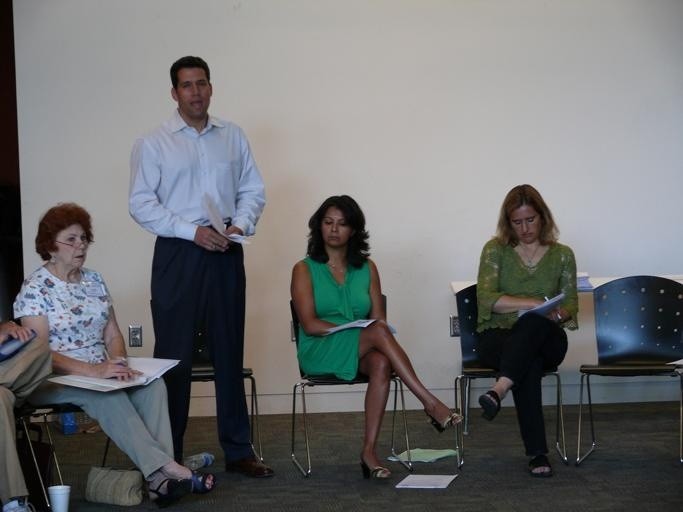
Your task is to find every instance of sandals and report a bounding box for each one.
[479,391,500,421]
[193,475,216,491]
[148,480,195,505]
[529,456,552,477]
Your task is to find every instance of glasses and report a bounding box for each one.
[53,237,94,251]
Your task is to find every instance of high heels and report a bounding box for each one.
[424,410,464,434]
[360,459,390,479]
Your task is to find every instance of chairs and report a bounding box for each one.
[454,283,568,467]
[577,276,683,464]
[150,299,263,462]
[291,294,414,476]
[6,404,112,508]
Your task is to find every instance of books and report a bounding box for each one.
[47,354,181,395]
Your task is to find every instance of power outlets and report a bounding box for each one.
[450,315,459,337]
[130,326,143,347]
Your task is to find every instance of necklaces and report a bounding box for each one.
[475,184,580,476]
[520,243,539,265]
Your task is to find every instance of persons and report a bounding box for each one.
[127,57,275,478]
[0,320,53,512]
[290,194,464,484]
[13,203,214,507]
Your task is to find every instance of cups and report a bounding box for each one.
[48,485,70,512]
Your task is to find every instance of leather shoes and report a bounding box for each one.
[227,458,275,478]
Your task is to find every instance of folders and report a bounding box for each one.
[0,328,38,363]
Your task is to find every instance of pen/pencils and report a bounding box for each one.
[544,296,564,320]
[104,349,112,360]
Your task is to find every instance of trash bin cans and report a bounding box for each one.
[51,413,101,436]
